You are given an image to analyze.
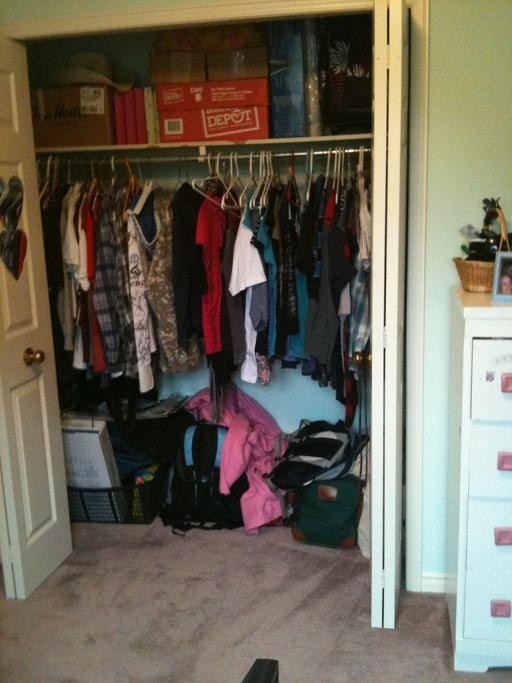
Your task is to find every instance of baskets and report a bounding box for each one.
[452,204,512,294]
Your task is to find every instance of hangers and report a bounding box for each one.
[333,144,367,191]
[286,150,300,207]
[39,156,53,200]
[66,159,72,185]
[121,156,161,221]
[239,152,285,210]
[306,148,314,202]
[323,147,331,193]
[89,157,119,186]
[167,153,243,219]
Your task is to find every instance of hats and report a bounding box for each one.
[53,52,134,91]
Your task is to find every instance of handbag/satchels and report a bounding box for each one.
[291,472,362,549]
[167,466,226,536]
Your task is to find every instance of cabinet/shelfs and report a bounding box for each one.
[452,289,512,674]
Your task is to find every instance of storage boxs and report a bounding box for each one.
[159,107,268,143]
[151,51,207,80]
[207,48,268,81]
[32,82,115,143]
[154,23,267,48]
[157,78,271,111]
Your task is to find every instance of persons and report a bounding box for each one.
[498,271,512,296]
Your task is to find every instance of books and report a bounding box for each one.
[113,86,159,145]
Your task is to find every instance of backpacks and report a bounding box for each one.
[171,424,249,534]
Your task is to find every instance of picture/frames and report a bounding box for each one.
[492,251,512,302]
